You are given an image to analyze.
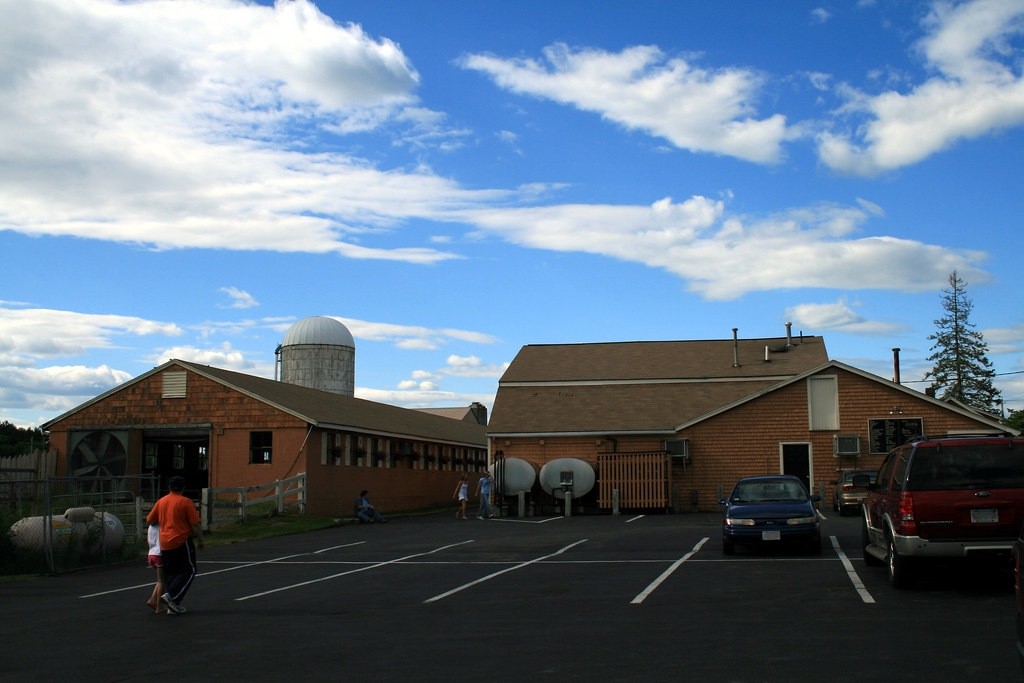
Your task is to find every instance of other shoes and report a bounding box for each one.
[456,512,459,518]
[462,516,468,519]
[382,521,388,524]
[489,514,493,517]
[477,516,485,520]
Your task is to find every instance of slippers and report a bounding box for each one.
[155,609,167,614]
[146,603,156,609]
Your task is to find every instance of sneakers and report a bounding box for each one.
[160,592,187,614]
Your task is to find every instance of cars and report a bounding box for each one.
[829,469,903,516]
[719,475,823,557]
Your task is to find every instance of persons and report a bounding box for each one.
[353,490,389,523]
[148,475,205,614]
[452,471,471,519]
[474,471,495,520]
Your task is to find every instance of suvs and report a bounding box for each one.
[861,429,1024,587]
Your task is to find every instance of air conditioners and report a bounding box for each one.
[834,435,862,454]
